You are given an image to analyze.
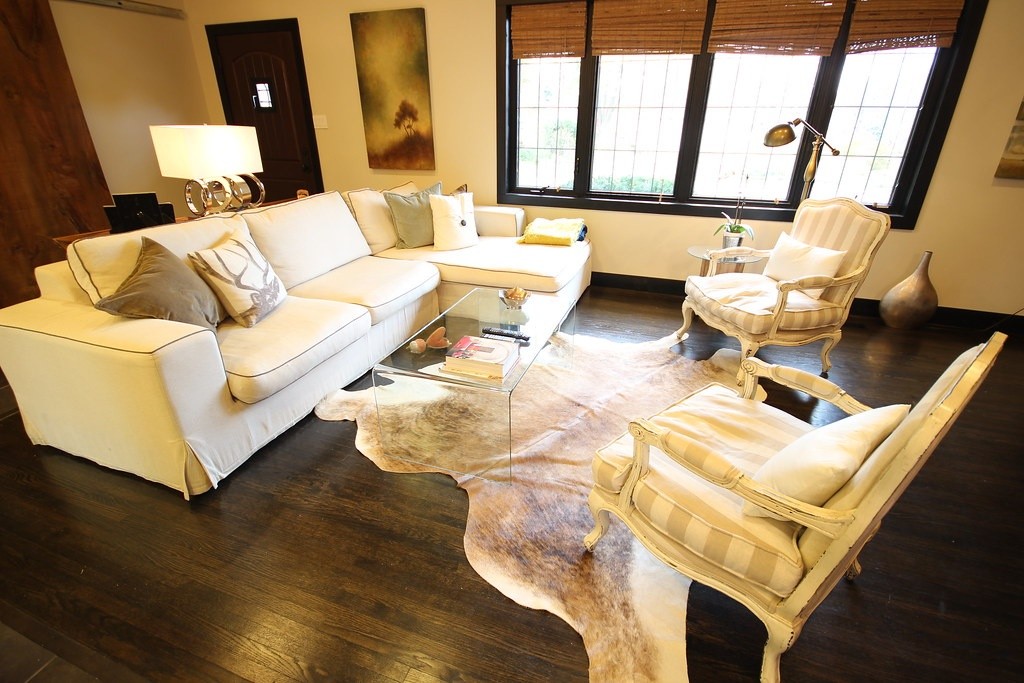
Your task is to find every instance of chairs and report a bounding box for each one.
[676,196,890,387]
[581,332,1008,683]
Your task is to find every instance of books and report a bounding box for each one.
[438,335,521,385]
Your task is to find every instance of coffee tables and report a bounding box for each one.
[371,287,576,488]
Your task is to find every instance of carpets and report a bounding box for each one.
[314,331,766,683]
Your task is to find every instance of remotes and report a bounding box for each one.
[480,333,516,343]
[482,327,531,341]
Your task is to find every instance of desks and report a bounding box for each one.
[50,198,299,259]
[684,247,762,320]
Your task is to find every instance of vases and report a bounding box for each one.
[880,251,939,329]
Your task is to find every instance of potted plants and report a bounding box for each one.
[714,212,754,248]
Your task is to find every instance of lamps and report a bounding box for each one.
[763,119,839,201]
[150,125,265,221]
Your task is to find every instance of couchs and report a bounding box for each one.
[1,182,592,501]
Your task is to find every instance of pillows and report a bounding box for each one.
[186,226,288,327]
[383,183,442,250]
[411,183,481,237]
[90,235,228,336]
[763,231,848,300]
[428,193,480,250]
[742,404,912,521]
